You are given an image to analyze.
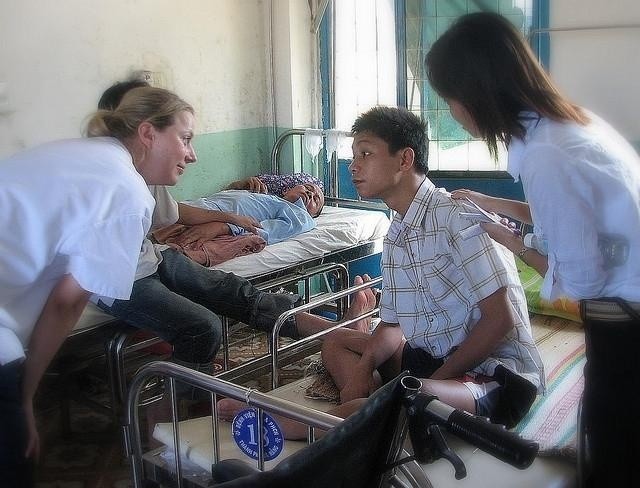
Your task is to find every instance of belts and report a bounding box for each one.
[581,299,640,321]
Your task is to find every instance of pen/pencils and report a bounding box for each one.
[491,211,522,235]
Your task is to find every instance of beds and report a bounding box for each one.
[124,198,589,488]
[26,130,395,433]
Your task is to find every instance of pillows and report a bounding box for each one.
[515,256,583,325]
[242,173,323,199]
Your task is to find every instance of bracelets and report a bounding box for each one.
[516,247,528,261]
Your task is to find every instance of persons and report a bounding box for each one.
[425,11,639,488]
[0,89,196,488]
[146,177,325,268]
[97,79,379,451]
[218,106,547,458]
